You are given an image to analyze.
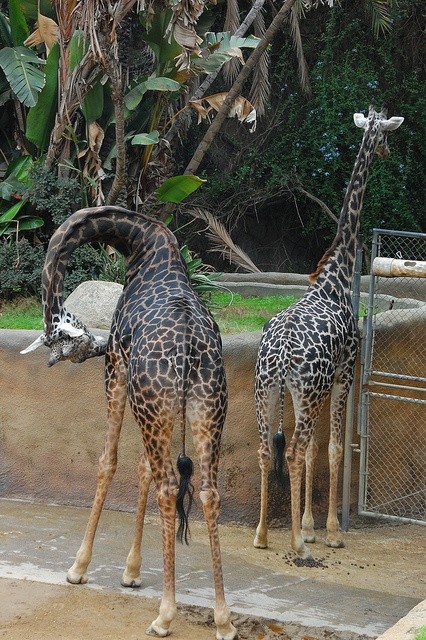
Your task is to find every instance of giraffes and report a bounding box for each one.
[253,103,406,560]
[19,205,241,640]
